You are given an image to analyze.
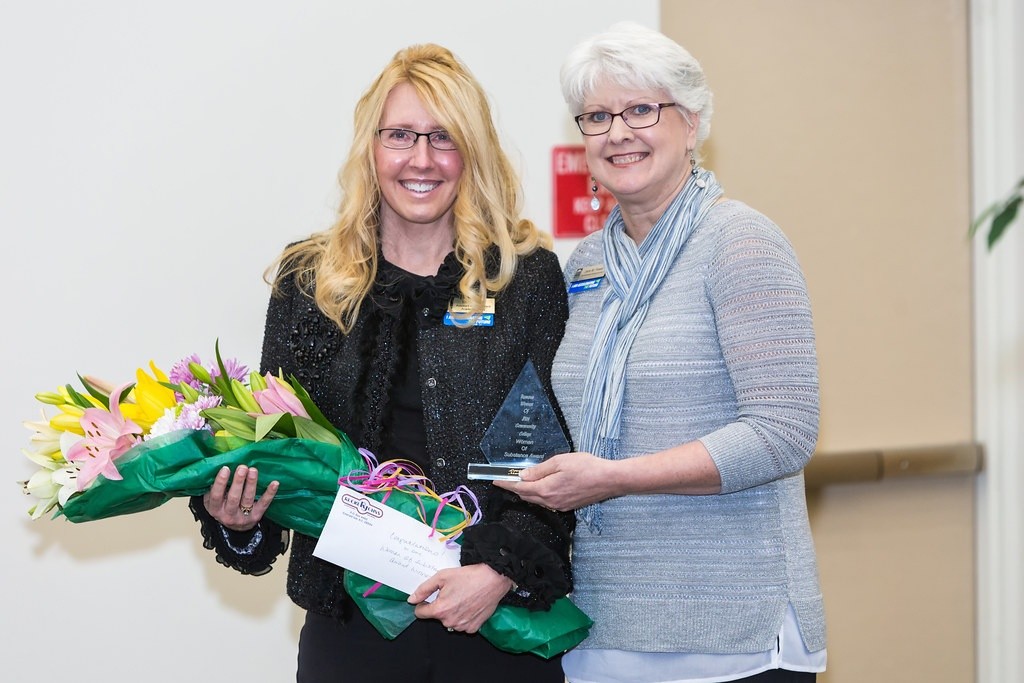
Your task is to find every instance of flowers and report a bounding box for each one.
[12,338,592,659]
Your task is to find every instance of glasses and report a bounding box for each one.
[575,101,676,136]
[374,128,459,151]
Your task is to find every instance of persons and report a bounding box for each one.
[496,24,826,683]
[187,44,576,683]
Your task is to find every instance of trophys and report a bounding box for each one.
[466,358,571,480]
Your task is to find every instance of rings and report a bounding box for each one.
[239,504,253,515]
